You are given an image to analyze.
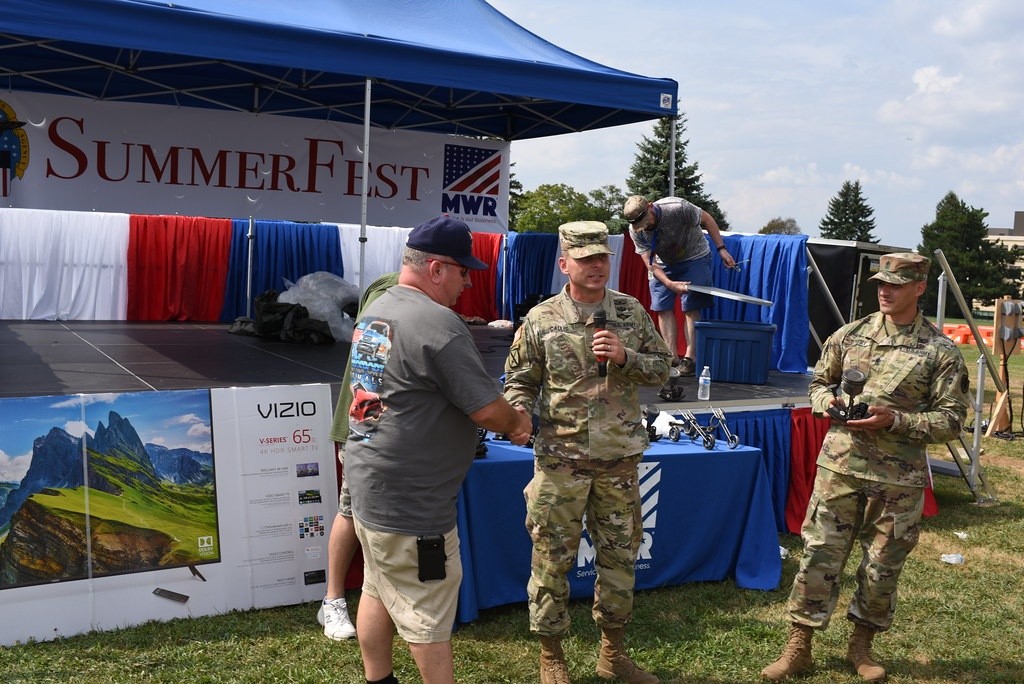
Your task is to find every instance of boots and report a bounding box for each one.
[761,624,812,683]
[540,635,571,684]
[596,628,661,684]
[847,624,886,682]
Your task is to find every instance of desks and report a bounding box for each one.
[452,431,783,631]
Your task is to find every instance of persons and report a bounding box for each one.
[343,212,533,684]
[316,271,401,644]
[505,220,670,684]
[623,195,735,376]
[762,251,970,684]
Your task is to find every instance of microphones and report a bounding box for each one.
[593,309,607,377]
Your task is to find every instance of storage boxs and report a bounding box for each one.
[694,319,778,385]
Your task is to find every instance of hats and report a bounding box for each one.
[405,213,489,269]
[624,195,650,229]
[867,252,931,284]
[558,221,615,258]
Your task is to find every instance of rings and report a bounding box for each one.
[608,345,610,351]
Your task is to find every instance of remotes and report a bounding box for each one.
[152,588,189,602]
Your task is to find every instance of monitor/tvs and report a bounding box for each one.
[0,387,222,581]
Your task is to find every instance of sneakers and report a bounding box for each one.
[676,357,696,376]
[671,358,679,367]
[316,596,356,640]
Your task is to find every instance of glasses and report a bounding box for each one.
[627,203,647,224]
[425,257,470,277]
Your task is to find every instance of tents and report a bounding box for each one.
[0,0,677,322]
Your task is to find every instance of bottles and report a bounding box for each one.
[698,366,711,400]
[941,554,965,564]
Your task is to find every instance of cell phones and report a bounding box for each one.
[417,534,447,583]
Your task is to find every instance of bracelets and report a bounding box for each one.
[715,246,729,253]
